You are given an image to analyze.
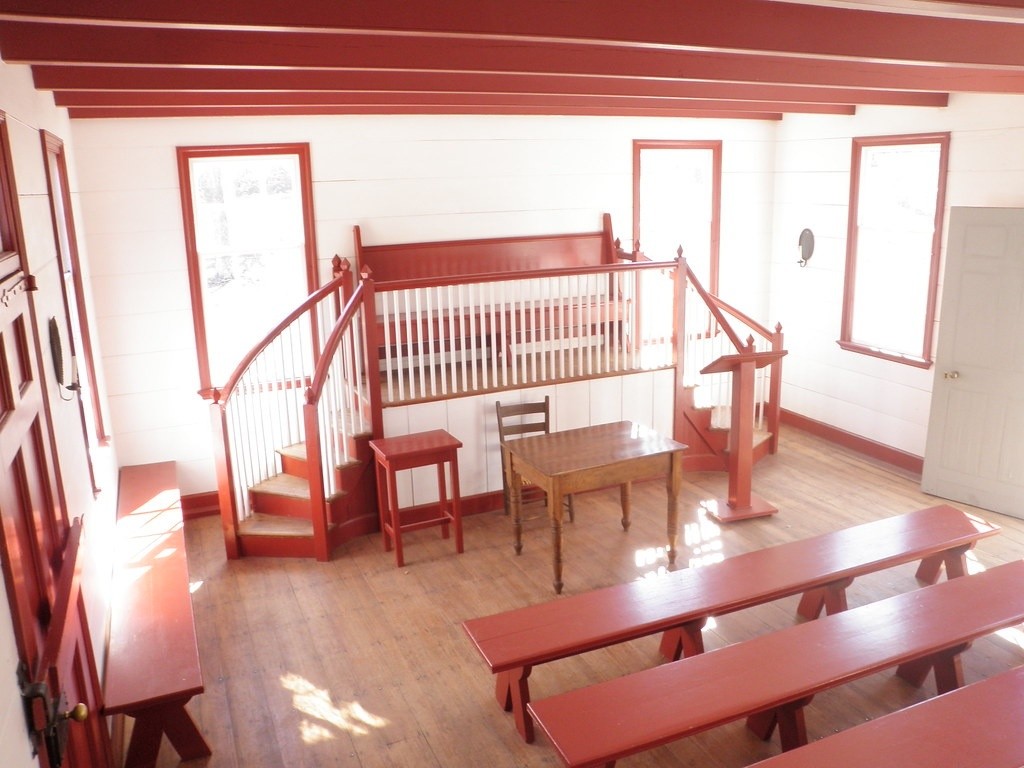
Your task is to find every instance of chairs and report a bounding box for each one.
[496,395,574,526]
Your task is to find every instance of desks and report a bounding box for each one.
[500,420,690,593]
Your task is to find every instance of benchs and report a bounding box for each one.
[104,461,214,768]
[462,502,1024,768]
[354,214,632,384]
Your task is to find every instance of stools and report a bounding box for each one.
[369,428,464,567]
